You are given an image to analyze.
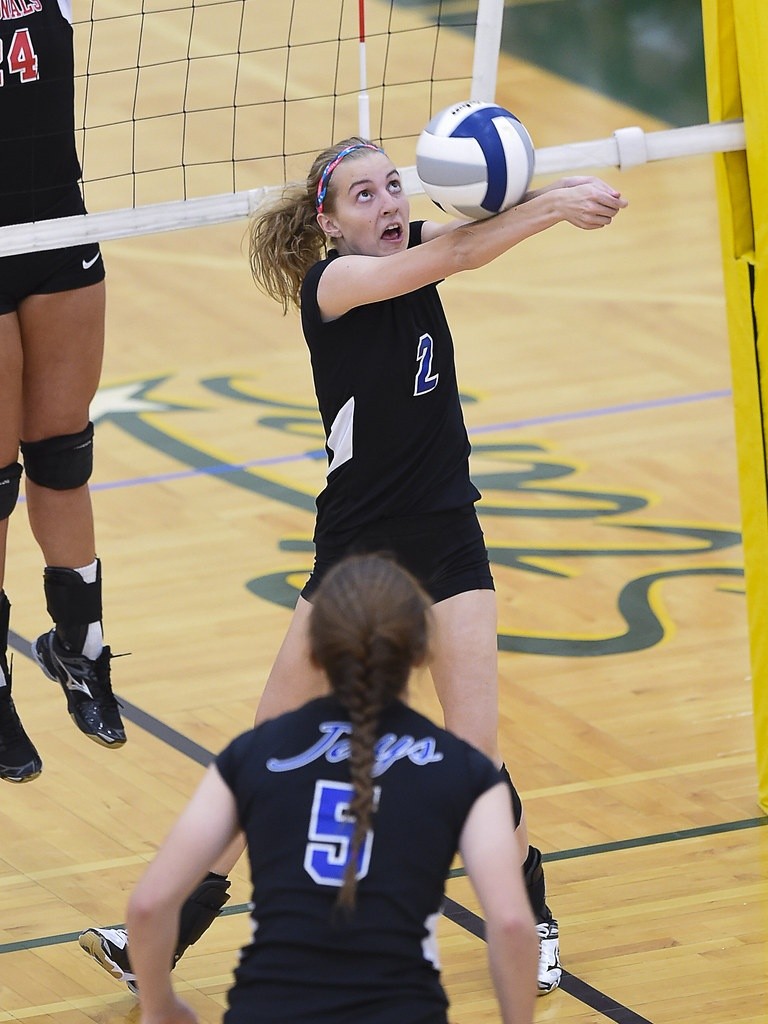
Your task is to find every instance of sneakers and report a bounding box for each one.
[535,904,561,995]
[31,628,127,749]
[0,653,43,784]
[79,926,175,1001]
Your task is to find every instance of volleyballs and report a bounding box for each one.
[416,101,535,220]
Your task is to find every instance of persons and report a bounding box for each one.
[76,134,629,997]
[124,558,541,1024]
[0,0,128,782]
